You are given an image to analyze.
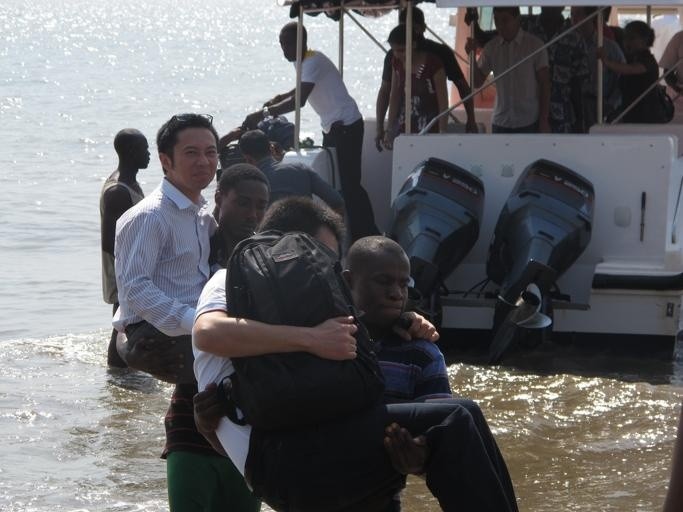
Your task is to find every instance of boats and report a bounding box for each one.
[275,1,682,367]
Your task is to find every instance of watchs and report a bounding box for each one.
[261,105,270,119]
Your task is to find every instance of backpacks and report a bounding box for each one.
[226,229,386,425]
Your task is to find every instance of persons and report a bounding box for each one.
[239,22,376,246]
[373,4,682,155]
[97,112,517,511]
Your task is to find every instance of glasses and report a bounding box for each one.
[160,114,213,141]
